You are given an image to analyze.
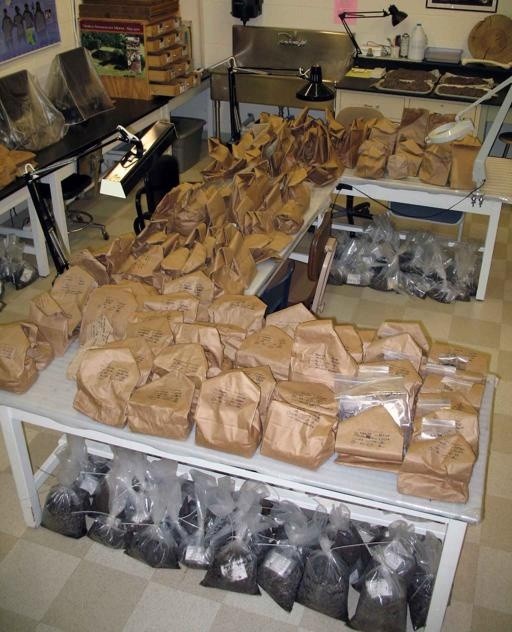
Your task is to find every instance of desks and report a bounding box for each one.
[1,69,213,277]
[334,165,503,301]
[242,164,340,311]
[333,60,511,147]
[0,329,495,631]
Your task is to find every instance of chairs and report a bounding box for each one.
[290,212,335,313]
[387,198,465,241]
[258,264,295,316]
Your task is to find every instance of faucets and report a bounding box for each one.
[276,31,308,48]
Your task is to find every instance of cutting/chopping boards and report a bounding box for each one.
[466,14,512,64]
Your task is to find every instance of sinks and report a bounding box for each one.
[205,58,347,111]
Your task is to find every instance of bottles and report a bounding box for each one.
[395,23,425,61]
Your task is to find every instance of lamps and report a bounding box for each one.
[227,58,336,147]
[18,119,179,276]
[338,2,409,65]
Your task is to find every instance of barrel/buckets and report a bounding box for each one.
[407,23,428,61]
